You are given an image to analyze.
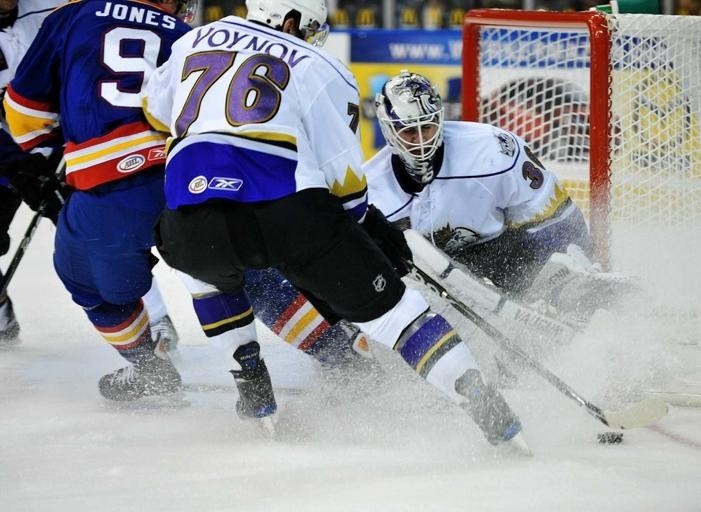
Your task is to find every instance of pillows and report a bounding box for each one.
[374,69,444,183]
[245,0,326,31]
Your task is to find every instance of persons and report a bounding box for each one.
[0,0,179,361]
[2,1,386,409]
[359,73,701,438]
[142,0,521,445]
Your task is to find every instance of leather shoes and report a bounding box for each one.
[363,204,413,278]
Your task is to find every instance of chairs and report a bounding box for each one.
[453,369,521,447]
[98,315,182,401]
[229,341,276,420]
